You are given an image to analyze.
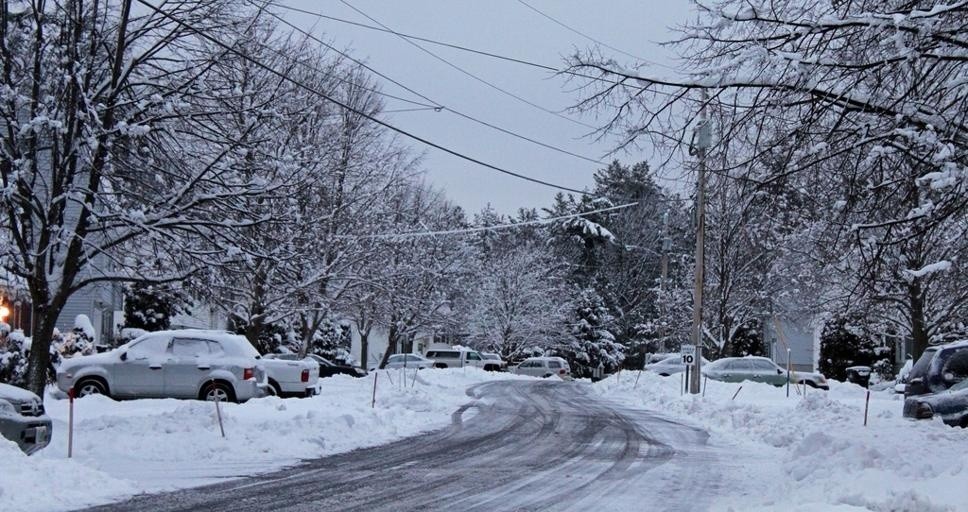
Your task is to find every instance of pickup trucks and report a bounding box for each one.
[425,347,505,371]
[261,346,322,401]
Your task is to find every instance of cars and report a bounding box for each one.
[644,348,708,379]
[363,350,438,373]
[688,355,832,395]
[0,377,54,460]
[53,325,260,406]
[508,356,571,384]
[309,352,356,379]
[899,340,968,428]
